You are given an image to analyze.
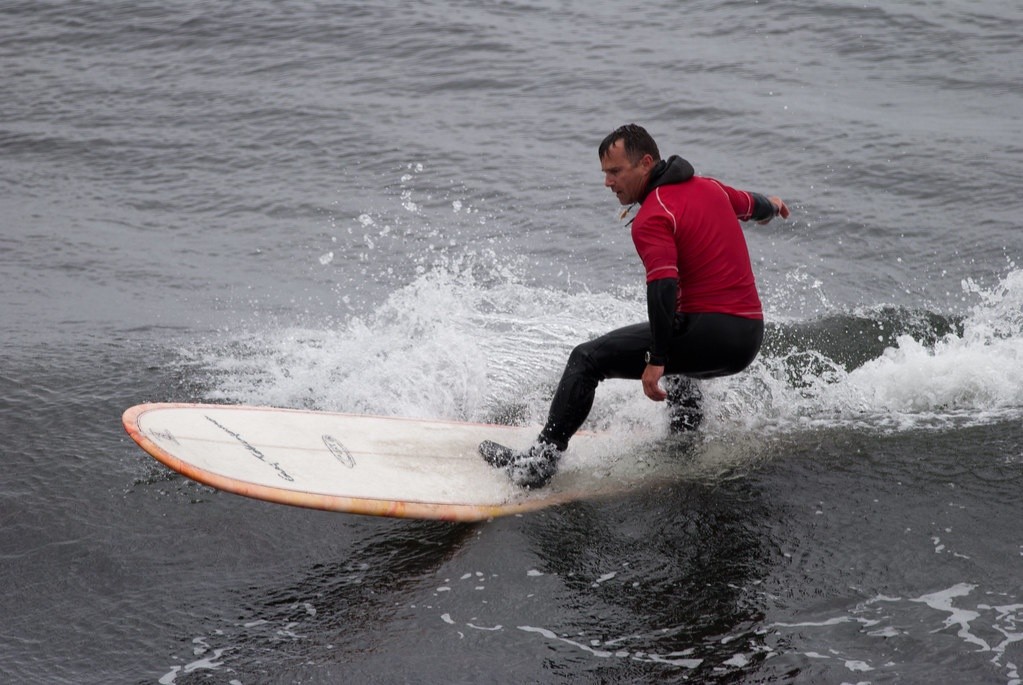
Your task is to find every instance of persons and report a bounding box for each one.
[476,122,791,489]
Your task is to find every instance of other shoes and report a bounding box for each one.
[666,385,704,447]
[479,440,550,490]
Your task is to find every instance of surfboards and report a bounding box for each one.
[120,400,761,522]
